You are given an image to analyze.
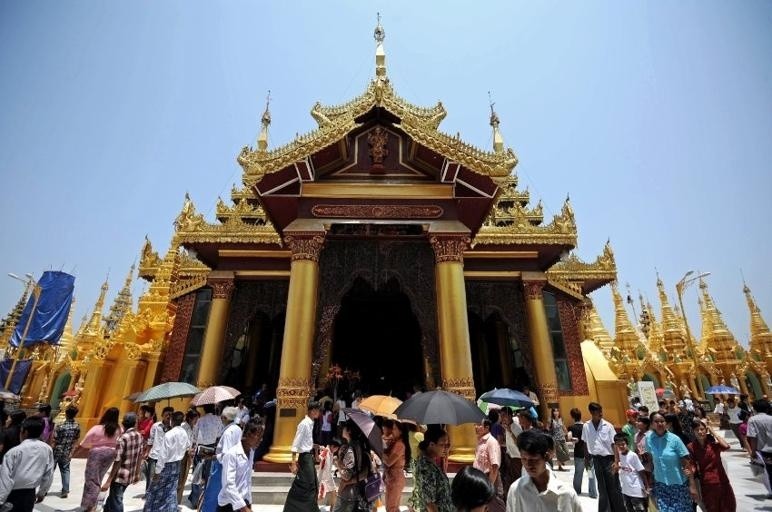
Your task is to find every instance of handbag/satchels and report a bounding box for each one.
[360,473,384,502]
[197,446,214,457]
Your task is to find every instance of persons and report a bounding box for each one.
[713,395,772,493]
[290,391,411,511]
[408,410,581,511]
[1,394,143,510]
[138,384,264,512]
[567,403,736,512]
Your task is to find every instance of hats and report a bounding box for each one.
[123,412,136,423]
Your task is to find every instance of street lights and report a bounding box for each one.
[676,267,712,400]
[1,269,44,393]
